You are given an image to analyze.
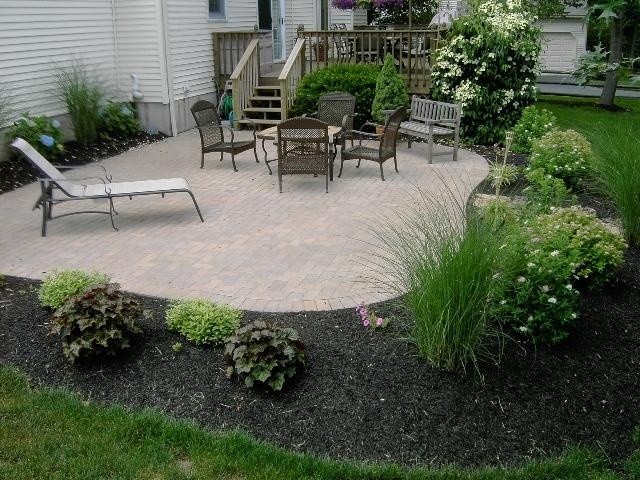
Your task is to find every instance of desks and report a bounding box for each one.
[255,124,342,175]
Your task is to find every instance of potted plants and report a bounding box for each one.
[369,53,409,142]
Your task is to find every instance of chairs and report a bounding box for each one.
[190,100,259,172]
[330,23,438,73]
[7,137,205,237]
[276,91,407,193]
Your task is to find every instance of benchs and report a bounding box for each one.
[395,95,464,163]
